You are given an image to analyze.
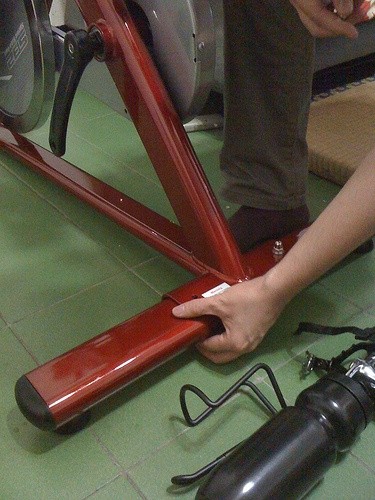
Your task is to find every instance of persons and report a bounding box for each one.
[220,1,375,257]
[171,1,375,365]
